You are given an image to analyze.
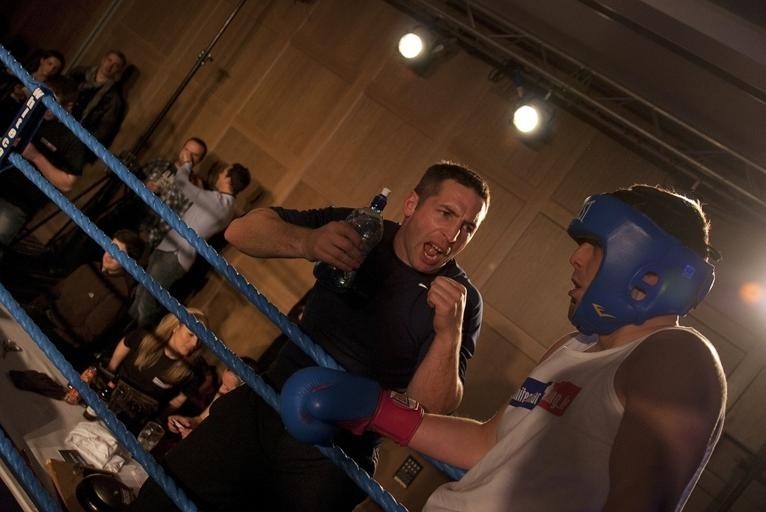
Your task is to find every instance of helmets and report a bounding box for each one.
[566,193,722,336]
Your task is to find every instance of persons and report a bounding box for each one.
[128,159,490,511]
[280,183,728,511]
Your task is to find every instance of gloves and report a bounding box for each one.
[279,367,425,445]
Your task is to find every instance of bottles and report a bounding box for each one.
[64,365,98,406]
[313,186,392,296]
[83,373,120,423]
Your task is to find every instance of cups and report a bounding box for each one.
[137,422,165,453]
